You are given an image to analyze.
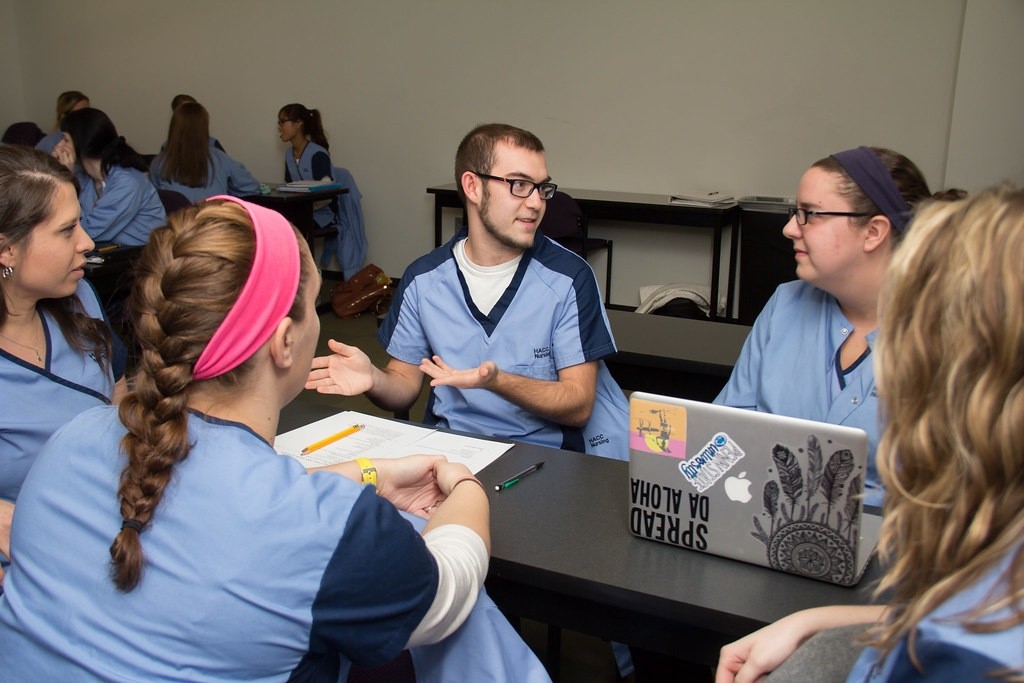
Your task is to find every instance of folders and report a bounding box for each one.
[276,180,342,193]
[737,196,797,211]
[668,191,734,208]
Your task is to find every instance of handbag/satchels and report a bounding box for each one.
[330,262,393,320]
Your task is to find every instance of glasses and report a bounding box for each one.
[787,207,884,225]
[468,170,557,200]
[277,119,297,125]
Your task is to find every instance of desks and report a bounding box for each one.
[242,183,349,316]
[272,412,896,683]
[83,241,147,276]
[426,184,799,403]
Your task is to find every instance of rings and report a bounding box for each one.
[426,505,433,513]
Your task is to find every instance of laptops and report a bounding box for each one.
[628,391,886,586]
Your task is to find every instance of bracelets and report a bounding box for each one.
[356,458,379,490]
[453,477,488,498]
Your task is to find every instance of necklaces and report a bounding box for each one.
[0,313,42,362]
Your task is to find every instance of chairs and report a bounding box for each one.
[539,190,613,308]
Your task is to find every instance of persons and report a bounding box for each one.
[0,193,489,683]
[305,122,631,465]
[0,88,90,154]
[0,144,118,593]
[49,106,169,339]
[150,94,262,208]
[710,189,1024,683]
[277,103,339,240]
[710,146,967,519]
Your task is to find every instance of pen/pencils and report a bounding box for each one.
[493,461,547,492]
[97,244,121,252]
[299,423,366,456]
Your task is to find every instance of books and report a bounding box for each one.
[276,179,341,192]
[268,406,515,478]
